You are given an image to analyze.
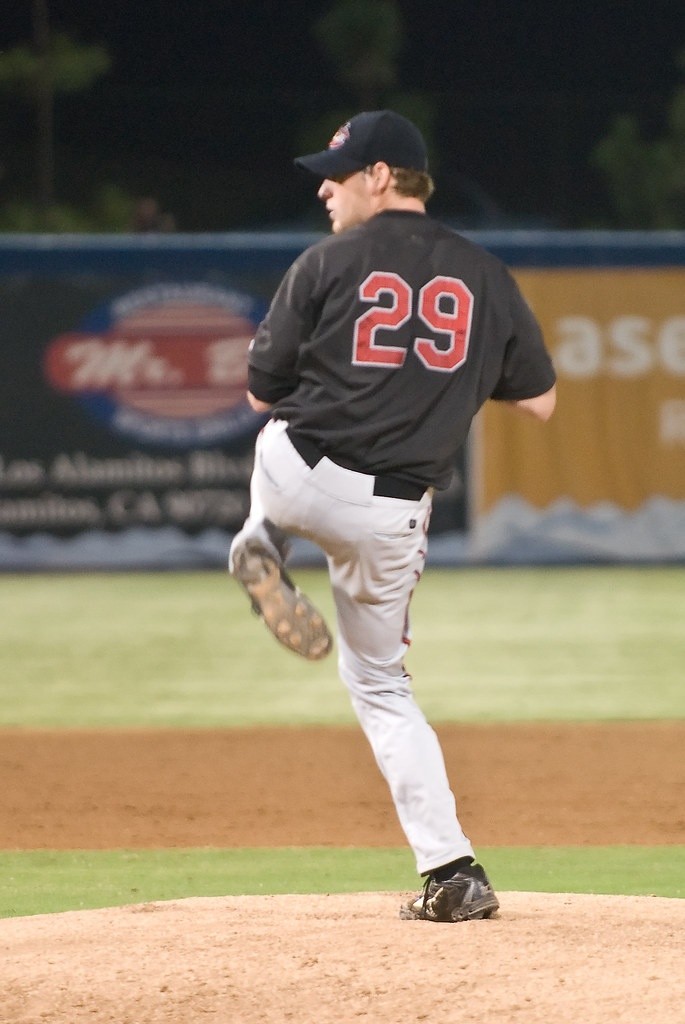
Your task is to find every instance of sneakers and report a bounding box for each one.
[400,860,500,922]
[231,539,333,660]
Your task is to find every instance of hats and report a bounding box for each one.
[292,111,429,182]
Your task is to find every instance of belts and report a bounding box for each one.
[285,426,426,502]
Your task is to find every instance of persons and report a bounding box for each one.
[227,111,559,922]
